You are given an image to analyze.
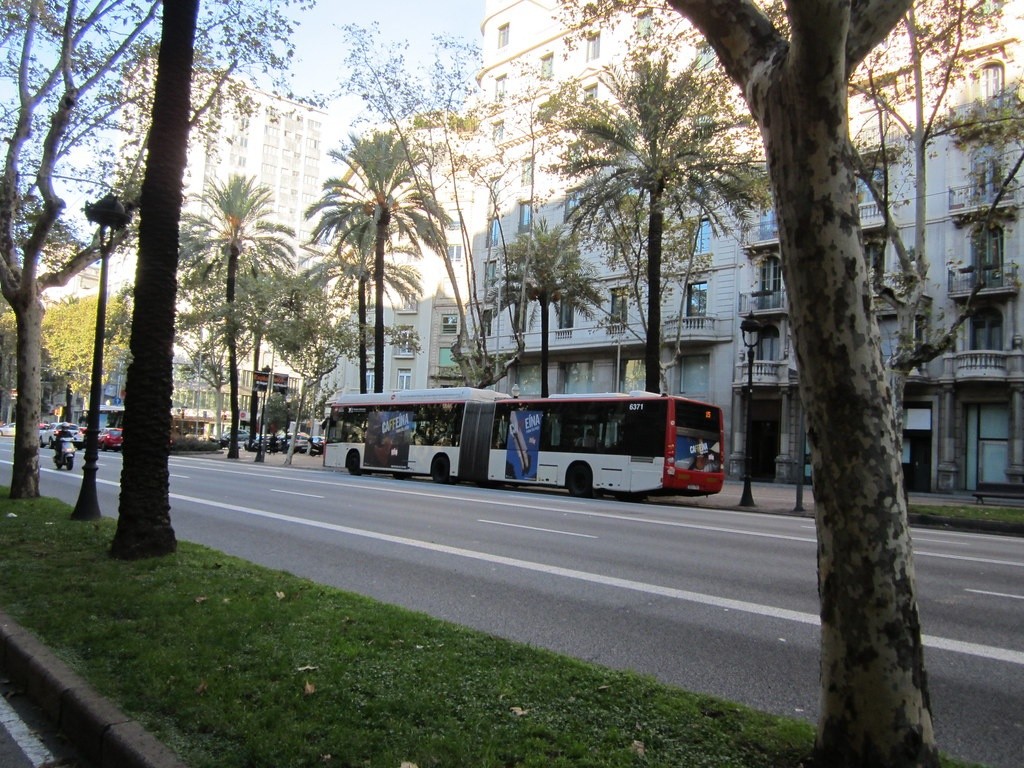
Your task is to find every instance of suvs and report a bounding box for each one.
[39,422,85,450]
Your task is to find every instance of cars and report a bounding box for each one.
[96,427,125,452]
[0,422,16,437]
[205,421,325,459]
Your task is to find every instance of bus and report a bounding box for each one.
[321,385,725,509]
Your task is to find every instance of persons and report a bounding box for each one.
[573,427,585,446]
[582,428,595,446]
[268,433,278,455]
[55,422,77,462]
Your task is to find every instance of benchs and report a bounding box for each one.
[971,492,1024,505]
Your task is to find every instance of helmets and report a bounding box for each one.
[61,423,70,431]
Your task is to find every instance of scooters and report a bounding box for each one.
[53,431,78,470]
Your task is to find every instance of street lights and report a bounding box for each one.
[62,191,135,520]
[735,309,765,509]
